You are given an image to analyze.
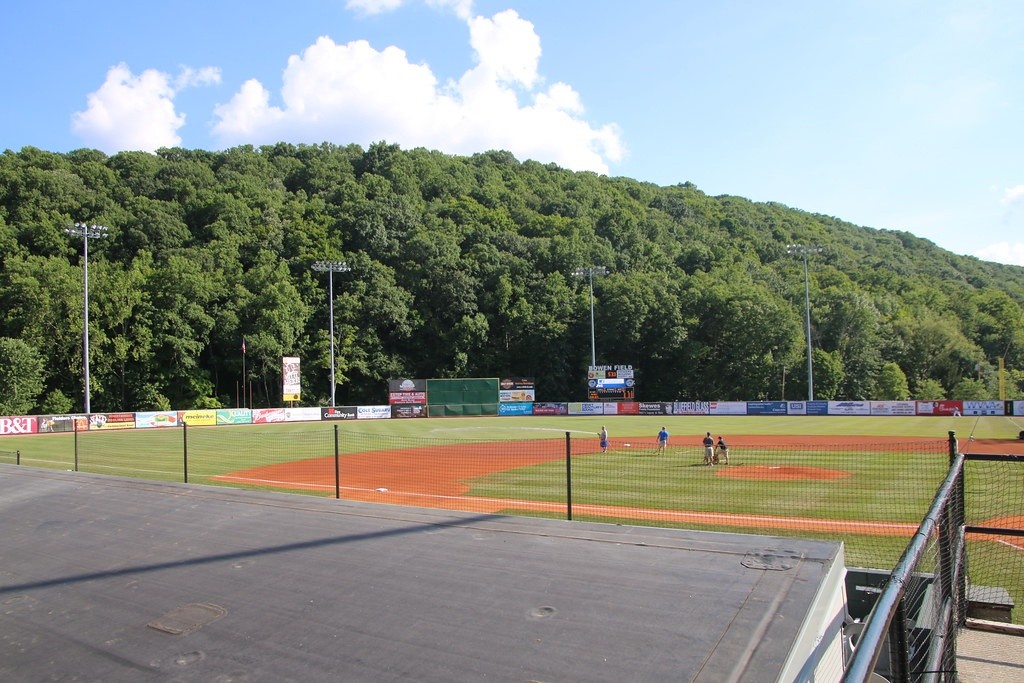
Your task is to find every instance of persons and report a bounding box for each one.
[702,431,729,466]
[656,426,669,455]
[599,425,608,453]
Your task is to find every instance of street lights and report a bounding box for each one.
[310,261,351,407]
[571,265,609,371]
[64,222,108,414]
[787,244,823,401]
